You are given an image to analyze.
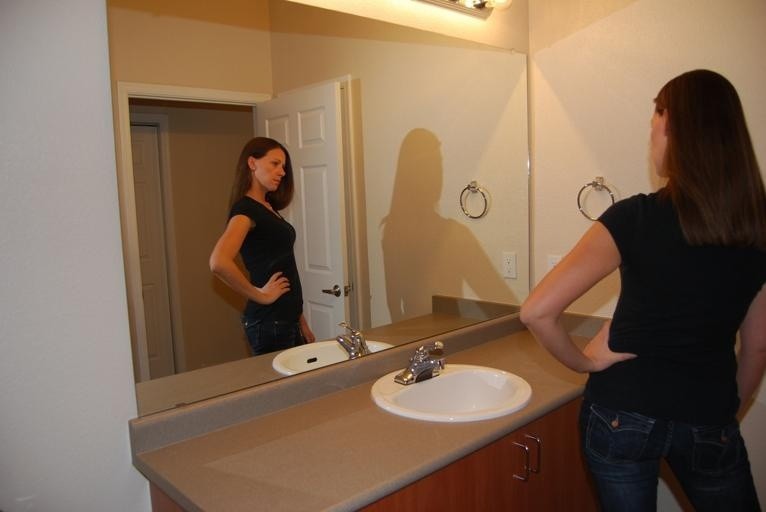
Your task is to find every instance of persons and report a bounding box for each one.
[517,72,765,510]
[210,135,317,362]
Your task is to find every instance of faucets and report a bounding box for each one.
[335,322,372,359]
[408,342,444,380]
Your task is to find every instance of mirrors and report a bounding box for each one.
[105,0,532,419]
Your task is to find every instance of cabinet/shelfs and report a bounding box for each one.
[358,392,585,512]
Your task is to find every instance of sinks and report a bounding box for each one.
[272,340,394,375]
[370,364,532,422]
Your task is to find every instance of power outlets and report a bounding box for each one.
[546,254,563,277]
[501,251,518,280]
